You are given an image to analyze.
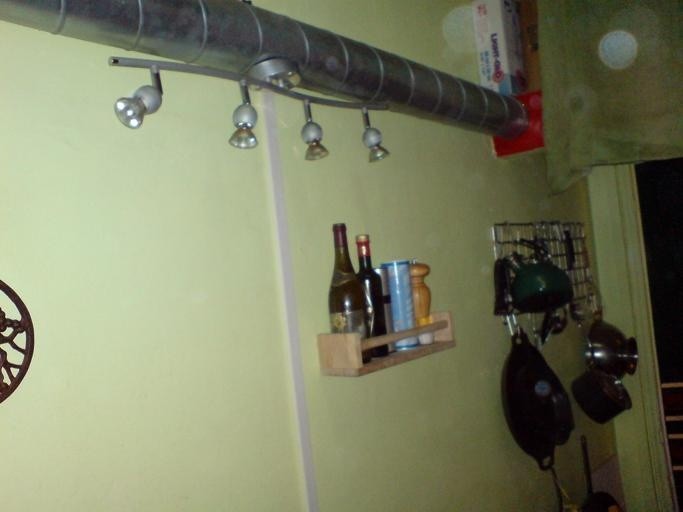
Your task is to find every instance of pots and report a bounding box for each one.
[547,433,623,511]
[487,257,572,473]
[562,320,644,429]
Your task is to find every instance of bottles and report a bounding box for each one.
[326,220,373,363]
[347,233,390,360]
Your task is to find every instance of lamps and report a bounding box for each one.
[107,55,391,163]
